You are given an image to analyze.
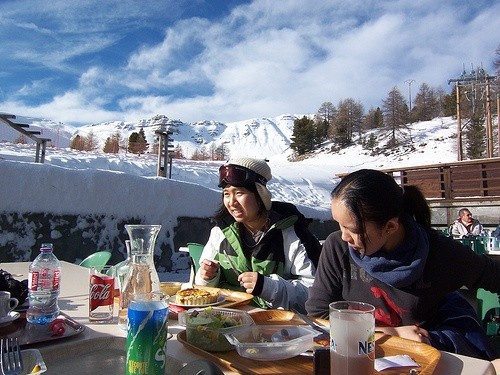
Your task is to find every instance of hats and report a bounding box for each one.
[219,157,273,211]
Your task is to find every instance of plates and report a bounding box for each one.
[271,326,330,356]
[0,348,47,375]
[0,311,20,327]
[167,285,254,314]
[168,294,225,307]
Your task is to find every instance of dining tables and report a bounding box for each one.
[0,262,497,375]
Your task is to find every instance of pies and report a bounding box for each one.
[159,283,181,295]
[175,288,219,305]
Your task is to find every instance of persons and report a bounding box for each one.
[452,208,488,240]
[306,168,500,362]
[195,158,323,315]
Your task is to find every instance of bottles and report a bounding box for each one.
[25,243,61,323]
[116,225,162,330]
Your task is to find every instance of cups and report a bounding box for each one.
[126,293,168,375]
[89,265,118,324]
[0,290,19,318]
[328,301,376,375]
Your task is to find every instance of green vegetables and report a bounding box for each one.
[185,306,247,352]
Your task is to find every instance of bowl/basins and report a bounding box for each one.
[229,325,315,361]
[179,307,249,351]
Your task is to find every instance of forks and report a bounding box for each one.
[0,338,23,375]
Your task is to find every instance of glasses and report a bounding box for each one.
[218,165,268,188]
[465,214,472,217]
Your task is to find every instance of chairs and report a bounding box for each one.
[473,286,500,361]
[79,251,112,273]
[438,227,495,255]
[186,243,203,273]
